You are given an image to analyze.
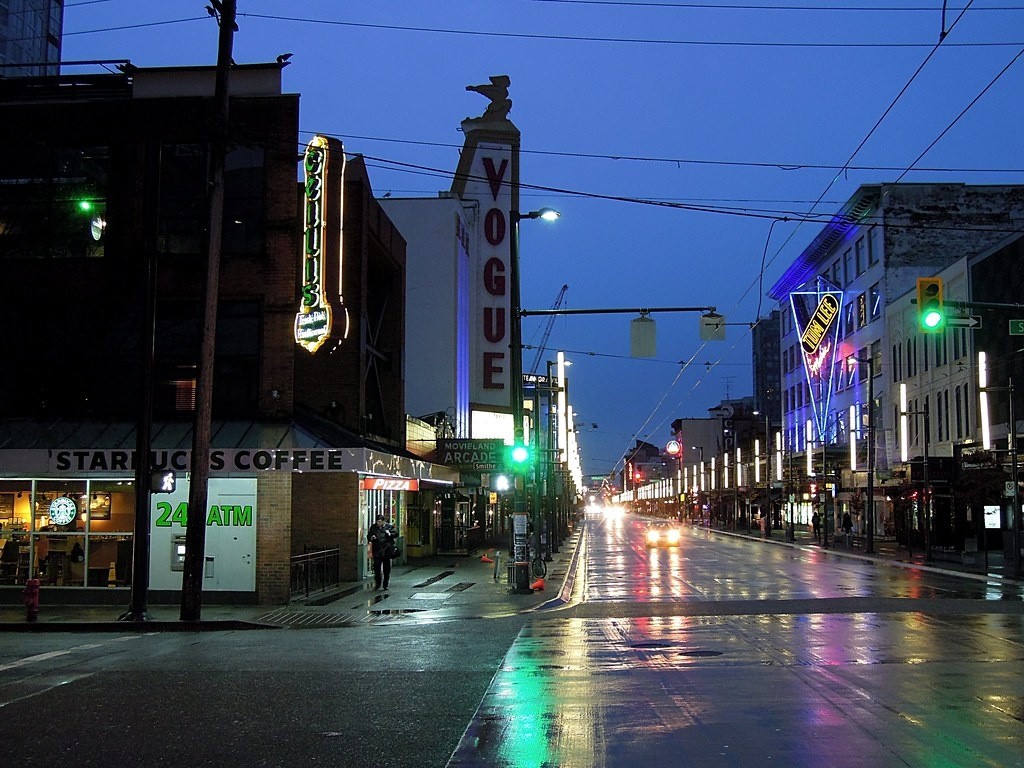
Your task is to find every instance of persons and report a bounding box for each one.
[366,515,399,591]
[812,512,821,538]
[0,529,19,575]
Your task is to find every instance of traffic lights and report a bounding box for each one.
[636,472,641,484]
[917,277,947,334]
[512,447,528,465]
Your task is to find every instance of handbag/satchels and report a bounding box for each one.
[386,546,401,559]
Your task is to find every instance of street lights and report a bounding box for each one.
[722,429,739,531]
[546,360,574,552]
[846,356,878,554]
[662,463,669,480]
[510,206,563,593]
[693,446,704,520]
[670,457,680,471]
[752,411,773,539]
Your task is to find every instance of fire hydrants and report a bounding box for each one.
[22,579,40,622]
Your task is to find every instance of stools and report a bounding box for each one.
[0,561,17,585]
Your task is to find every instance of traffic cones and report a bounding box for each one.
[479,556,494,563]
[529,578,546,593]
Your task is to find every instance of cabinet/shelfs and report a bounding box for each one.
[47,535,78,586]
[15,546,39,585]
